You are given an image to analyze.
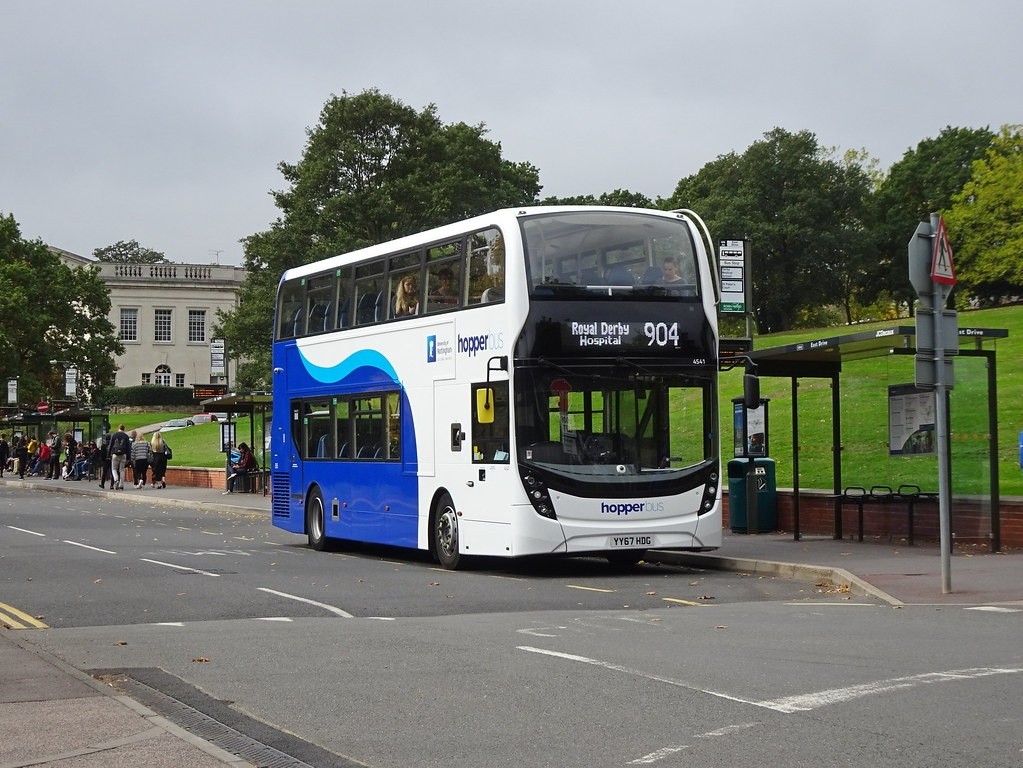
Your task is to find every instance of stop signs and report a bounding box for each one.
[37,401,49,414]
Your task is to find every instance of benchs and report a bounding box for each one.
[826,484,940,546]
[236,467,271,495]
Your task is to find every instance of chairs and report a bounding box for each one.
[582,266,663,294]
[285,285,504,338]
[317,431,386,459]
[569,430,630,464]
[503,426,546,456]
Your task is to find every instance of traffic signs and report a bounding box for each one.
[561,435,577,456]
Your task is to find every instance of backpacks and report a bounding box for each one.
[113,434,124,454]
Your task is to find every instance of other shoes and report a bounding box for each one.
[138,480,143,489]
[162,481,165,488]
[115,480,119,490]
[110,486,113,489]
[227,473,237,481]
[99,484,104,489]
[221,490,233,495]
[6,469,82,481]
[0,475,3,478]
[150,484,155,488]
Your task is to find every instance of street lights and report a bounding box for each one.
[49,359,81,428]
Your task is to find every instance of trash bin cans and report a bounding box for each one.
[726,457,777,534]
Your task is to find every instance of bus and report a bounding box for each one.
[269,201,760,570]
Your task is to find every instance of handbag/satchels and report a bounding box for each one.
[145,442,154,463]
[162,440,171,460]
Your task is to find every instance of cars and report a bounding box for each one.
[192,414,218,426]
[209,412,237,421]
[159,418,195,433]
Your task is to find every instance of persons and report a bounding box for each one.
[653,257,688,297]
[0,430,139,489]
[107,424,130,490]
[749,433,764,453]
[222,442,257,495]
[431,269,460,302]
[130,432,151,489]
[395,276,421,316]
[488,270,504,300]
[150,432,170,488]
